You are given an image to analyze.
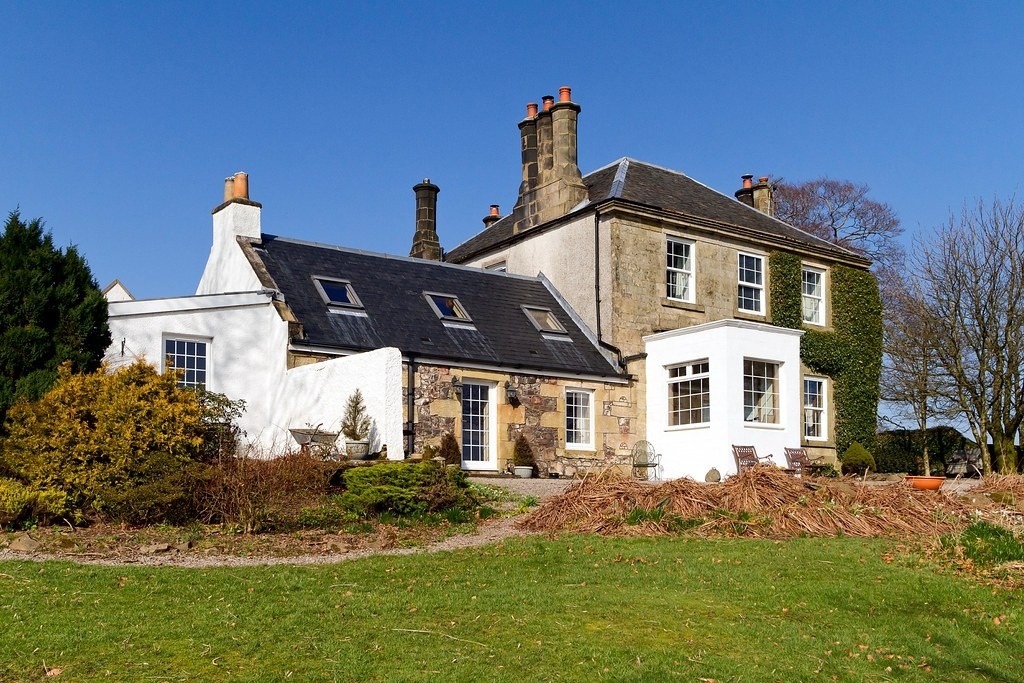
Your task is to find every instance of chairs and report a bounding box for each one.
[732,445,773,472]
[629,440,662,482]
[784,447,827,476]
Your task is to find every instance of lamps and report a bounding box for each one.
[505,381,518,404]
[451,375,463,394]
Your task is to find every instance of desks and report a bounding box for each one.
[778,469,796,476]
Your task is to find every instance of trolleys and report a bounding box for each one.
[288,423,346,459]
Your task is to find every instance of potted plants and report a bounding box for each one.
[440,431,461,467]
[513,431,534,479]
[342,387,372,460]
